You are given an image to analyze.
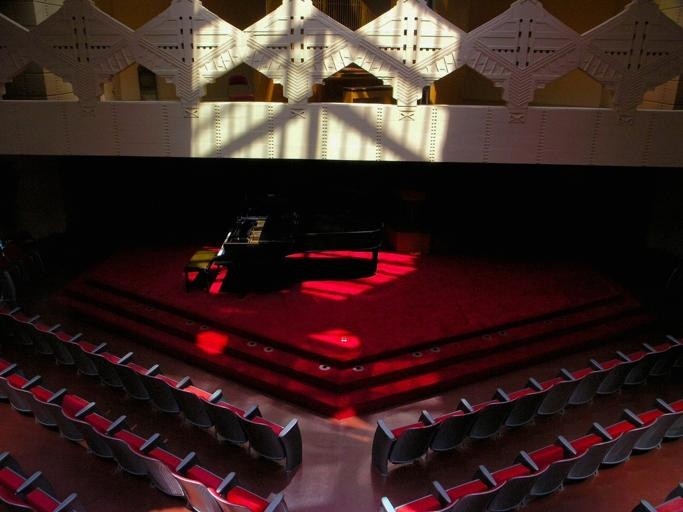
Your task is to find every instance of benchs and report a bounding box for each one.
[341,85,392,104]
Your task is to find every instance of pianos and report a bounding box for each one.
[215,213,382,297]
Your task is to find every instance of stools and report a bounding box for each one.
[185,250,218,293]
